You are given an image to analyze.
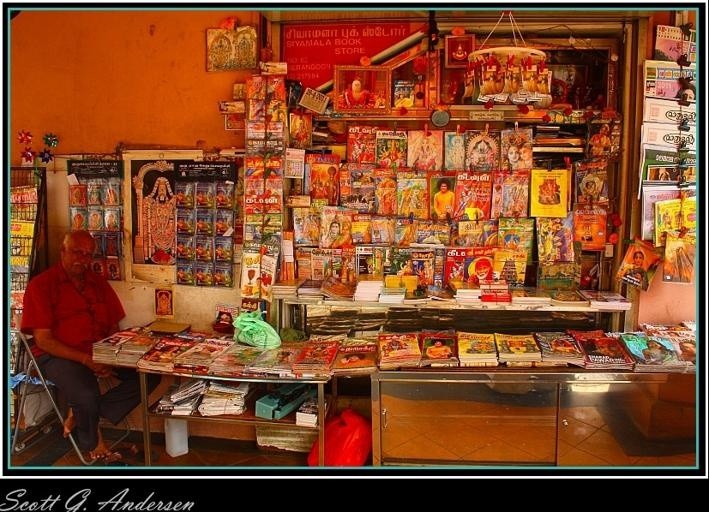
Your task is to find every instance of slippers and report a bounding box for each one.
[81,445,159,466]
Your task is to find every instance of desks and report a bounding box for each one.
[277,284,628,333]
[93,321,378,466]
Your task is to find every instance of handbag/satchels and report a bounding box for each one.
[229,310,284,349]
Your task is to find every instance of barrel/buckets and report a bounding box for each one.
[164,419,189,458]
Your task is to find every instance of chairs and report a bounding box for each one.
[9,318,131,465]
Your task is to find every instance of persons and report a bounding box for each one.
[19,229,161,459]
[94,80,699,428]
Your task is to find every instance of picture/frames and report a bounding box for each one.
[333,65,391,116]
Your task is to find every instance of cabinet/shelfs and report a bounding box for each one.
[445,35,476,69]
